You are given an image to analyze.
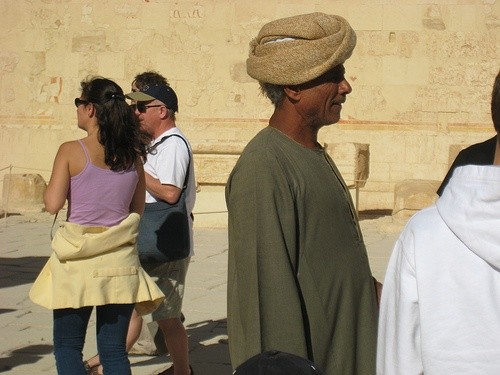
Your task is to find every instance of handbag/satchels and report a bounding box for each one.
[135,134,191,264]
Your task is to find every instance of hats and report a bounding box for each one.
[233,350,322,375]
[246,12,357,86]
[125,83,179,111]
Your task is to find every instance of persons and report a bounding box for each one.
[29,75,166,375]
[78,71,196,375]
[224,11,500,375]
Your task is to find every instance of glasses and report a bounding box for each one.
[130,104,172,113]
[75,98,95,107]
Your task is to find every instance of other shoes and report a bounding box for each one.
[83,360,103,375]
[157,364,193,375]
